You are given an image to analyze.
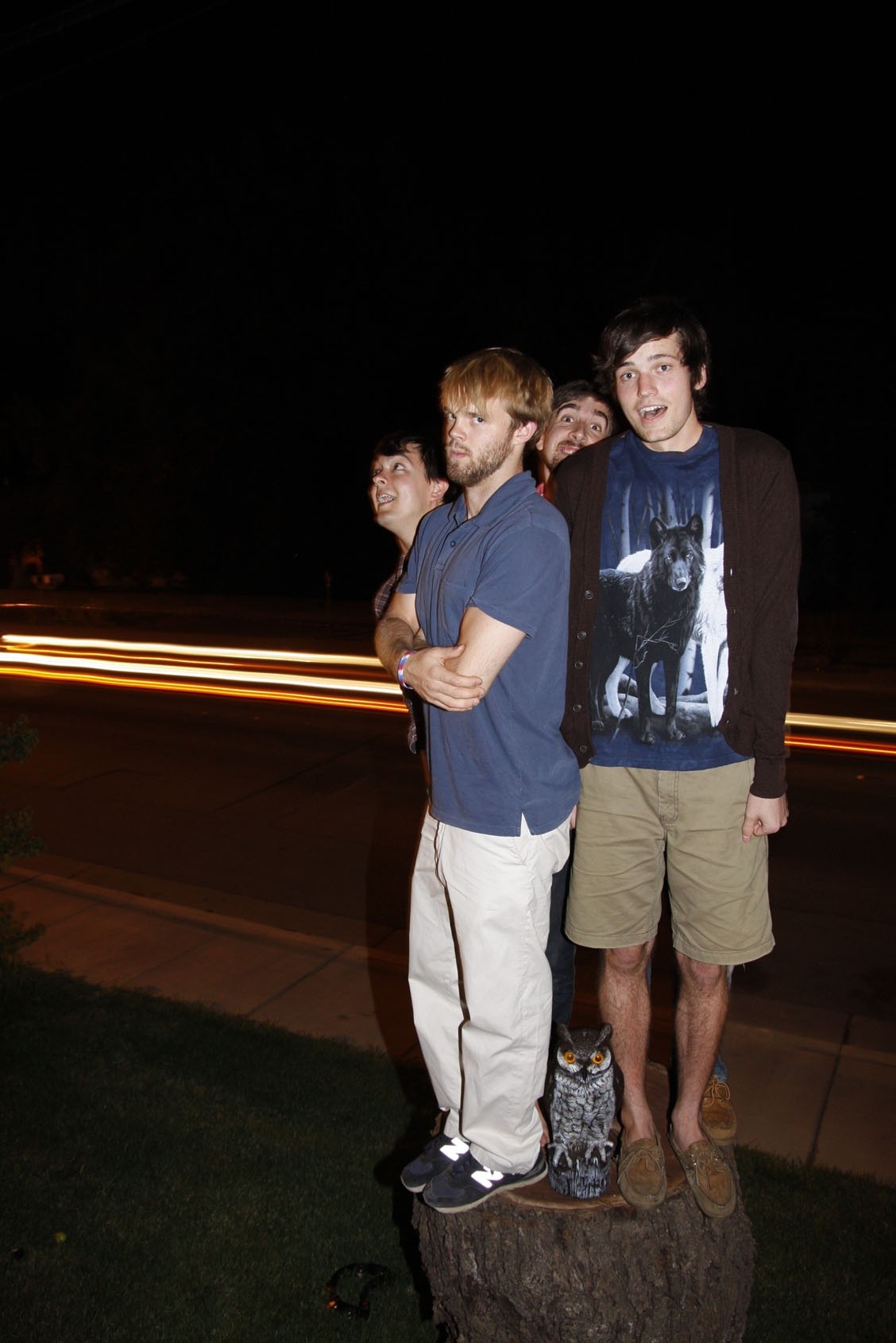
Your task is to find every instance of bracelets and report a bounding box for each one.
[397,650,416,689]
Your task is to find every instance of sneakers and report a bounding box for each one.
[400,1136,472,1192]
[422,1146,549,1214]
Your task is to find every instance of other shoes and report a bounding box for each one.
[668,1124,735,1220]
[697,1074,736,1146]
[618,1122,667,1209]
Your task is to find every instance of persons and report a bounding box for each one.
[371,350,580,1214]
[534,313,794,1220]
